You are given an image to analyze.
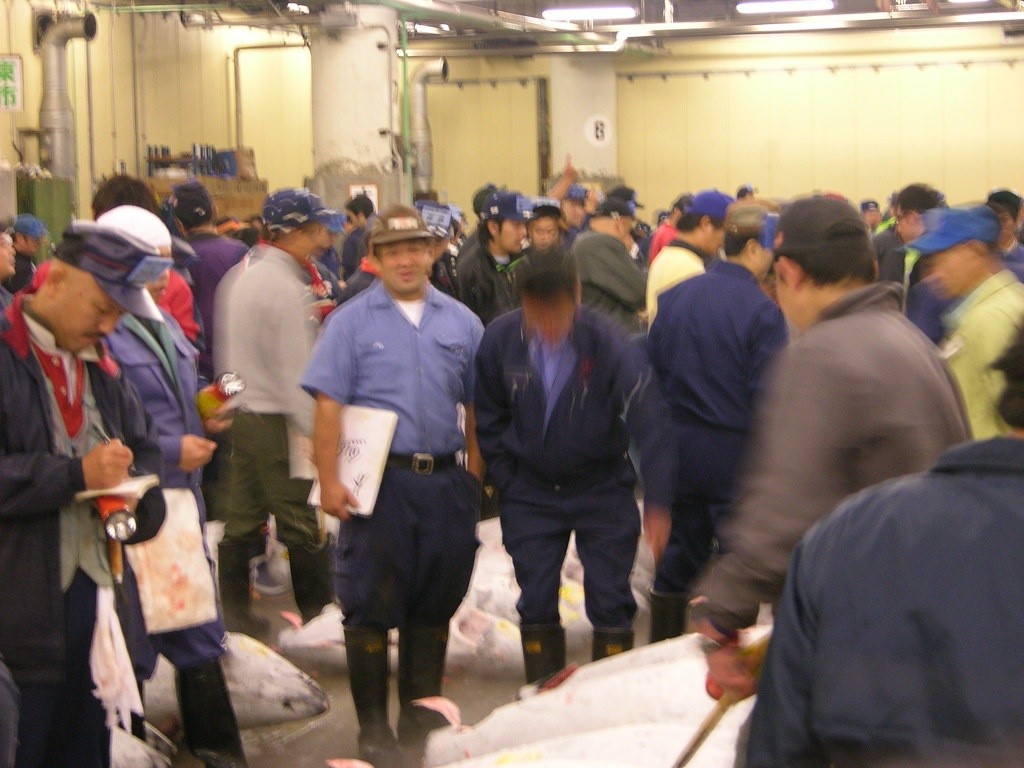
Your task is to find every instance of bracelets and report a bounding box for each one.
[702,638,729,653]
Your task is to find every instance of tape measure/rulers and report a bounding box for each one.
[675,626,774,766]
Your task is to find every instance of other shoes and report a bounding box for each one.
[590,626,635,664]
[523,623,566,690]
[652,588,690,641]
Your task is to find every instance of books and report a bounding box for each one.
[74,474,160,503]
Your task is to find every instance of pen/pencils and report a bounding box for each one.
[91,424,137,472]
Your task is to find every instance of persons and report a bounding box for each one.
[90,174,1024,636]
[644,198,789,644]
[301,204,485,768]
[735,315,1023,767]
[474,246,678,684]
[96,205,248,768]
[0,215,48,311]
[0,218,165,768]
[690,194,973,689]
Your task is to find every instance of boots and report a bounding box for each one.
[345,621,402,768]
[289,534,334,626]
[217,542,271,638]
[397,625,450,743]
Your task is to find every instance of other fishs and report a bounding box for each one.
[142,632,329,730]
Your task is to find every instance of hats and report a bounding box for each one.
[565,186,586,202]
[608,188,644,208]
[527,197,561,219]
[727,202,771,239]
[604,199,635,217]
[14,214,47,238]
[686,190,733,219]
[52,206,174,323]
[262,190,328,232]
[861,202,877,212]
[907,205,999,253]
[370,206,429,245]
[480,193,530,222]
[415,202,453,237]
[168,182,214,228]
